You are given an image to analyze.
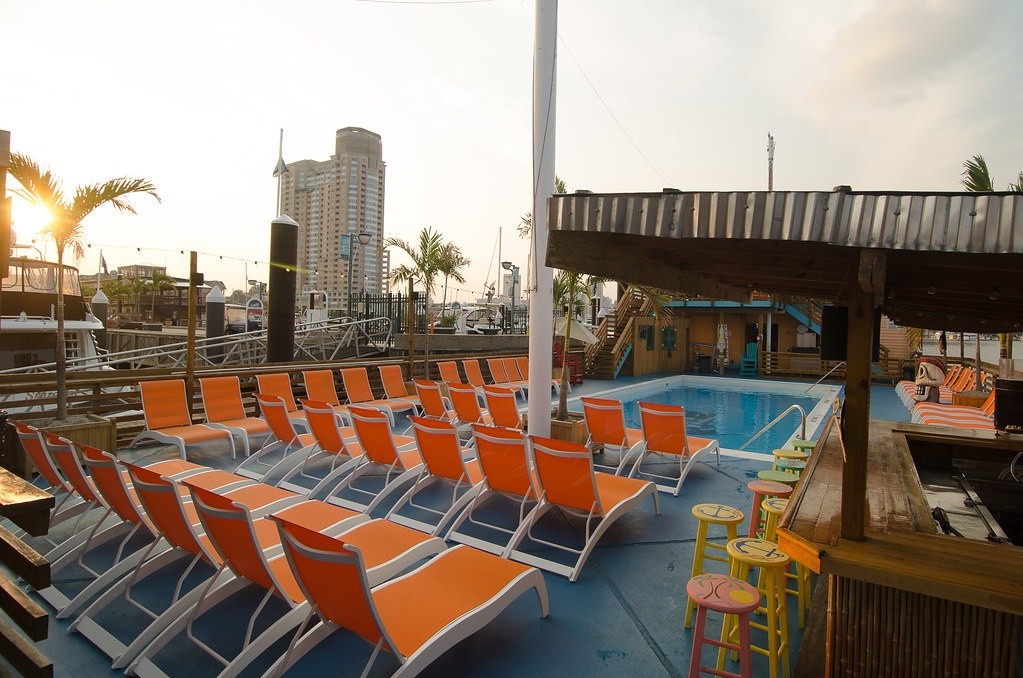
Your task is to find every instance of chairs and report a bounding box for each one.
[741,341,758,370]
[896,361,1004,428]
[5,341,714,678]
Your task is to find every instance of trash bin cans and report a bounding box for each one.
[141,324,162,331]
[118,321,142,330]
[699,356,711,374]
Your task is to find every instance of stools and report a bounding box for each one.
[791,439,818,455]
[755,470,800,539]
[772,450,808,461]
[774,459,809,473]
[684,573,761,678]
[745,480,794,572]
[759,499,813,629]
[714,538,796,678]
[683,503,747,630]
